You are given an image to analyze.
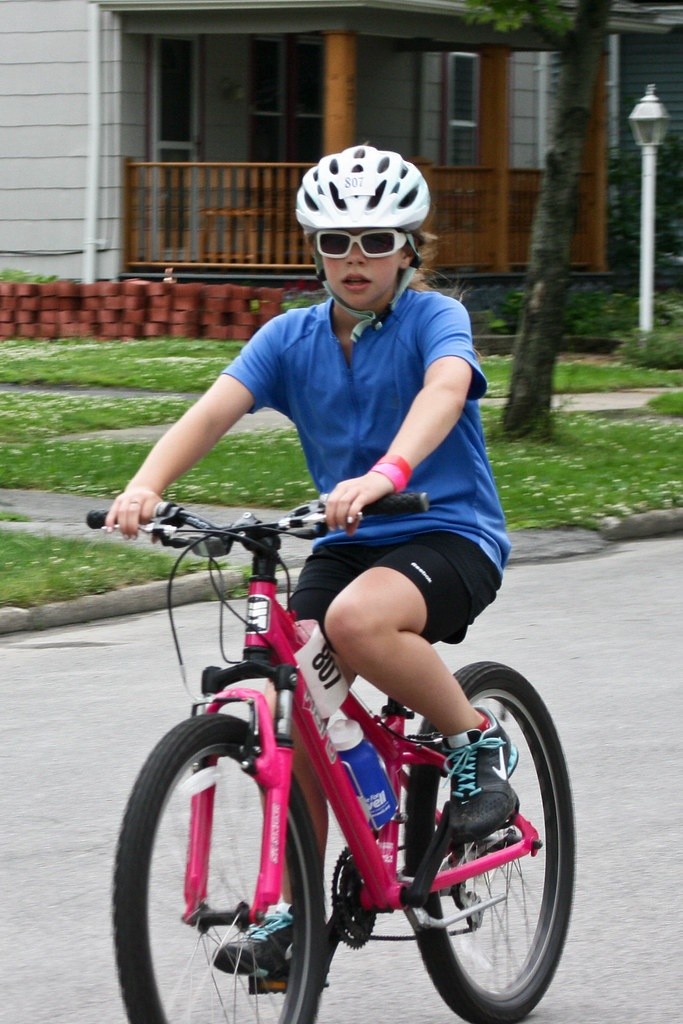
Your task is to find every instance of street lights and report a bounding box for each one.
[627,83,671,349]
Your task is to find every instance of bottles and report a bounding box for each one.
[325,713,398,830]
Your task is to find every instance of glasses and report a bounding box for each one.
[310,228,406,258]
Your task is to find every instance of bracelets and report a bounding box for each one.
[376,454,414,482]
[370,463,408,494]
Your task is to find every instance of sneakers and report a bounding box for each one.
[440,704,519,845]
[211,900,330,986]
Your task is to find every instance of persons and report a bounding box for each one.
[96,174,523,981]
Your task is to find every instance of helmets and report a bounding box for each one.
[294,144,431,230]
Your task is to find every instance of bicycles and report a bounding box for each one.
[86,491,574,1024]
